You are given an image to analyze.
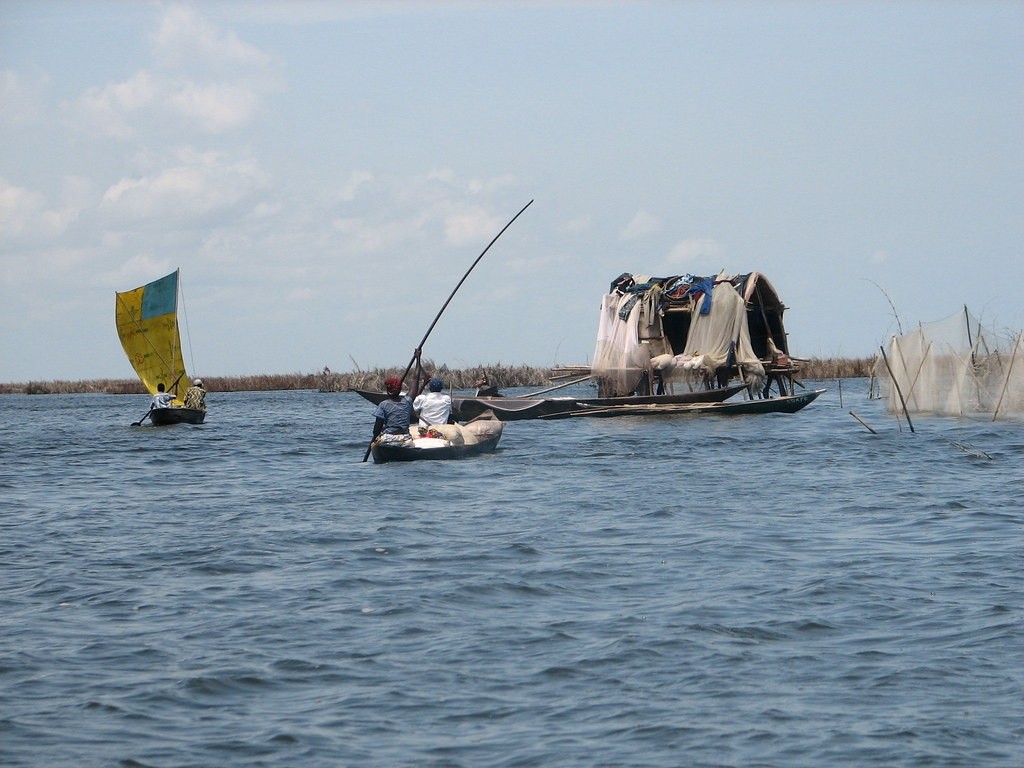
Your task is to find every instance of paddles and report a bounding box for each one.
[130,369,185,426]
[362,197,536,462]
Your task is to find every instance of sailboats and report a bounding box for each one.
[114,266,208,428]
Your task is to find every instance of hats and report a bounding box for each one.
[384,377,401,392]
[429,378,443,391]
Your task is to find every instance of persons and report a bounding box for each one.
[413,376,452,439]
[183,379,207,410]
[150,381,179,409]
[371,348,422,449]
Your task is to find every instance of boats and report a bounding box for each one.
[368,421,511,465]
[349,272,828,423]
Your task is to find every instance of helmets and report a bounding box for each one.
[193,379,202,385]
